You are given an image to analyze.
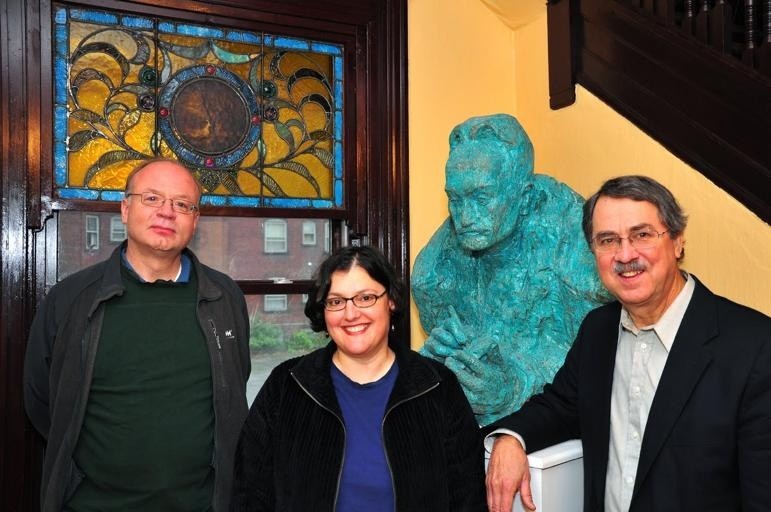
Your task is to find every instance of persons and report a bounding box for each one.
[229,244,485,512]
[22,157,252,510]
[481,175,771,512]
[413,113,618,427]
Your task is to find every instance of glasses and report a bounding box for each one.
[128,191,199,218]
[589,228,671,253]
[324,290,389,312]
